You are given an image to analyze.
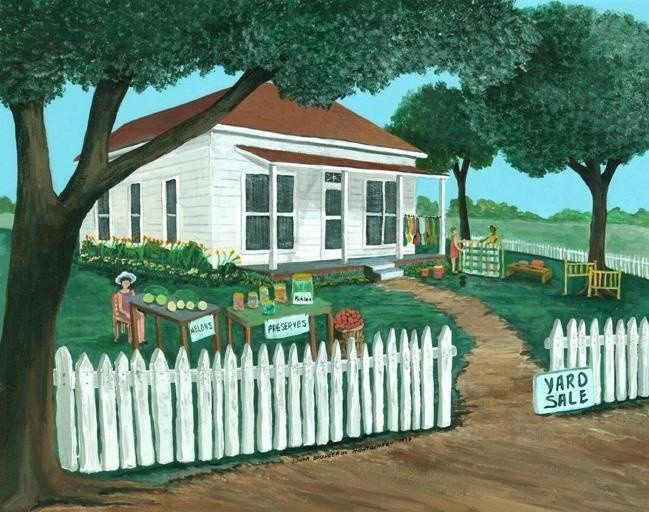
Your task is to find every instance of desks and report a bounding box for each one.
[508,263,550,284]
[127,291,334,363]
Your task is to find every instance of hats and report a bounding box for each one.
[114,271,138,286]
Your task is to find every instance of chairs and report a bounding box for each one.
[110,291,134,344]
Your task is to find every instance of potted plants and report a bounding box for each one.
[421,260,444,279]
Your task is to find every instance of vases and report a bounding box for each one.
[337,325,364,358]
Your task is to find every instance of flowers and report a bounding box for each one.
[332,307,363,331]
